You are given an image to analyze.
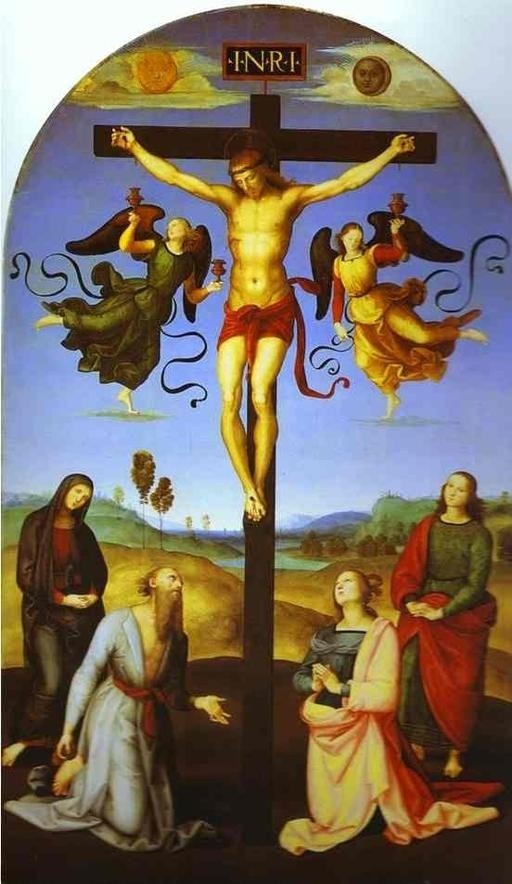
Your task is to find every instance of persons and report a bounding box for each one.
[33,207,225,416]
[5,470,108,766]
[288,564,439,834]
[329,214,493,424]
[107,121,419,525]
[49,562,234,836]
[388,470,497,780]
